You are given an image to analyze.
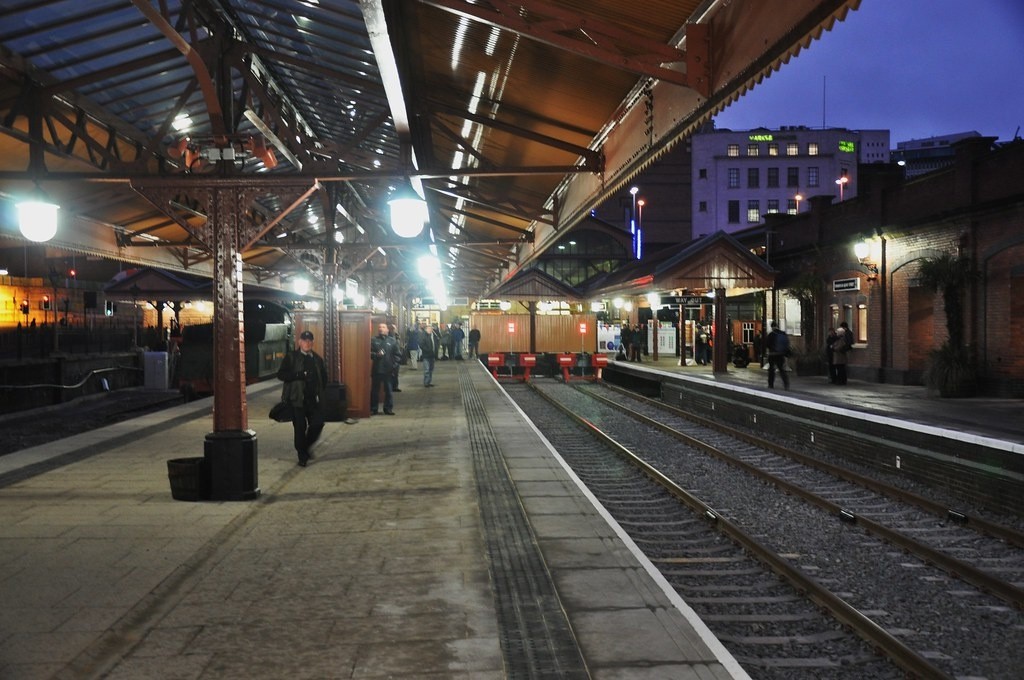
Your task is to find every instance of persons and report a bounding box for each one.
[417,324,440,387]
[385,324,403,392]
[404,320,482,371]
[826,321,855,386]
[11,311,184,355]
[763,320,791,391]
[370,323,396,417]
[618,322,647,363]
[276,332,329,466]
[694,322,764,370]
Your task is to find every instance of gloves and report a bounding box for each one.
[303,370,314,378]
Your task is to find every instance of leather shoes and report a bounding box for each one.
[370,410,378,415]
[384,410,395,415]
[299,457,307,466]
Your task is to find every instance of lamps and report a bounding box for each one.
[854,238,870,264]
[500,291,675,312]
[385,132,433,241]
[14,179,65,244]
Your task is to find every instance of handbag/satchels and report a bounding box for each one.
[269,402,294,423]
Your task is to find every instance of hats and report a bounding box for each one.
[771,321,777,327]
[300,331,314,340]
